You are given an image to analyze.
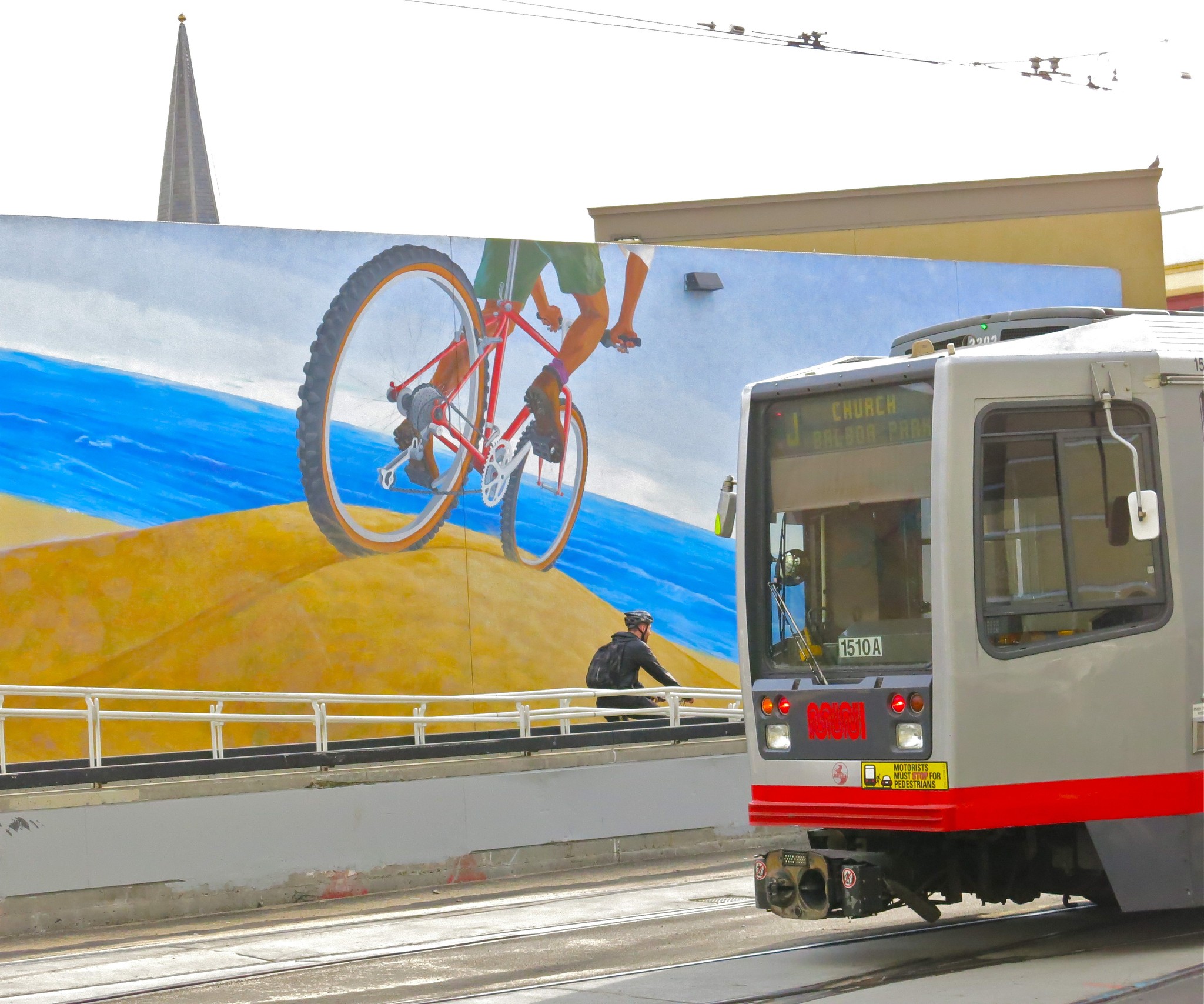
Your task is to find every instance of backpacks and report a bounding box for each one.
[585,637,639,690]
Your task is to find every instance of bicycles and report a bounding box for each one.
[610,697,693,721]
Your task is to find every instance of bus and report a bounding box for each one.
[713,306,1204,936]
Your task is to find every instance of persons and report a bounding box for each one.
[597,611,695,722]
[393,238,655,489]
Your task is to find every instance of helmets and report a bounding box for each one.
[624,611,654,629]
